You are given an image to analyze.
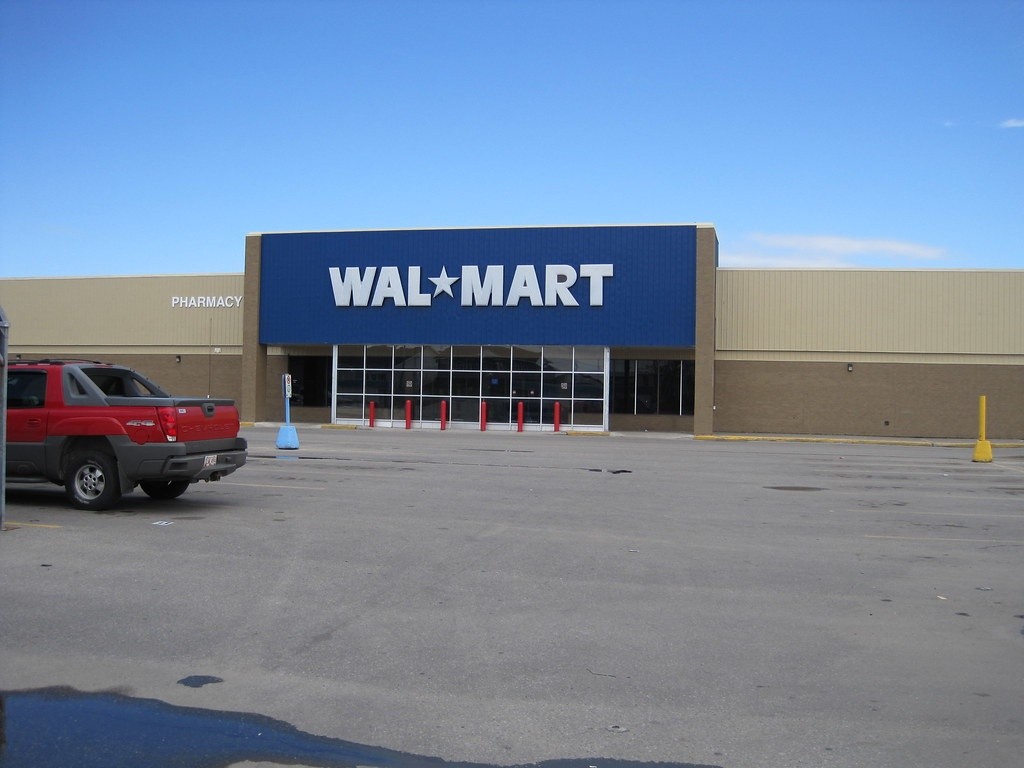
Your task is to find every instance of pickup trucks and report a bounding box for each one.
[5,359,248,511]
